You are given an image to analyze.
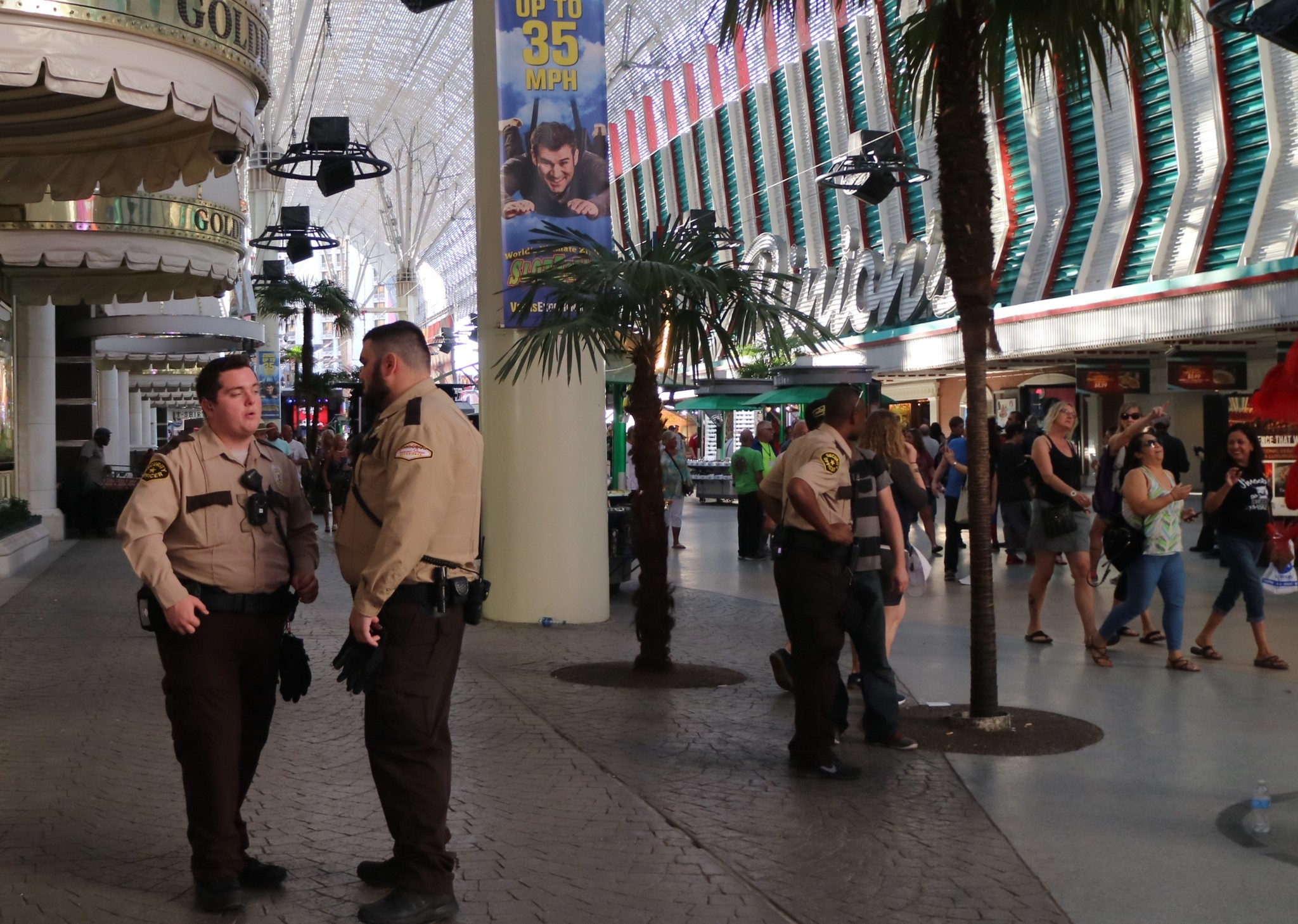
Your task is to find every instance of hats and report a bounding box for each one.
[1152,417,1170,426]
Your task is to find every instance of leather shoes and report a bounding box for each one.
[356,885,459,924]
[239,857,287,886]
[195,885,246,912]
[356,857,402,885]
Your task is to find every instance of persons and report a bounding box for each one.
[254,417,353,532]
[78,427,116,538]
[1024,400,1119,648]
[801,397,917,750]
[605,424,700,551]
[117,360,322,917]
[902,409,1067,581]
[770,638,794,693]
[1092,401,1169,642]
[1190,422,1290,669]
[259,380,280,399]
[1083,431,1201,672]
[725,405,808,561]
[328,319,486,923]
[847,409,928,706]
[756,384,869,781]
[498,117,611,221]
[1089,413,1226,584]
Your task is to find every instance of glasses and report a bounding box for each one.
[761,427,773,431]
[1119,412,1144,420]
[1139,439,1163,448]
[843,383,864,408]
[1059,409,1075,417]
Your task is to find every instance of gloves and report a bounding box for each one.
[279,633,311,703]
[333,628,386,695]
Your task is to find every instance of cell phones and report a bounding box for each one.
[1185,511,1205,520]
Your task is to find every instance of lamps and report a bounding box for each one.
[814,0,931,204]
[250,171,291,293]
[453,311,477,344]
[676,0,744,265]
[427,327,465,353]
[248,73,343,249]
[265,2,392,197]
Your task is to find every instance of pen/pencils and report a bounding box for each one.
[286,617,291,633]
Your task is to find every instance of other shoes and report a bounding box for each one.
[770,649,923,778]
[332,523,338,532]
[1189,546,1209,552]
[325,525,330,532]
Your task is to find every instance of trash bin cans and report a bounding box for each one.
[606,492,638,598]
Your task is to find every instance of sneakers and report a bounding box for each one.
[737,531,1066,581]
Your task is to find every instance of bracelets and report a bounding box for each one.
[910,462,919,472]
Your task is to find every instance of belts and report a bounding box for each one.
[201,593,291,614]
[350,584,468,604]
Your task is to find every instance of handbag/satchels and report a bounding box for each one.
[1264,524,1296,574]
[1103,518,1150,571]
[1039,502,1078,539]
[681,481,690,496]
[954,473,969,523]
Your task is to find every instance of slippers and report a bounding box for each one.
[1025,625,1289,672]
[671,544,685,549]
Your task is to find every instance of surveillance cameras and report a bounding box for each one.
[213,290,224,298]
[209,130,245,165]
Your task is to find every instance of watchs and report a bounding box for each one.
[1069,490,1078,499]
